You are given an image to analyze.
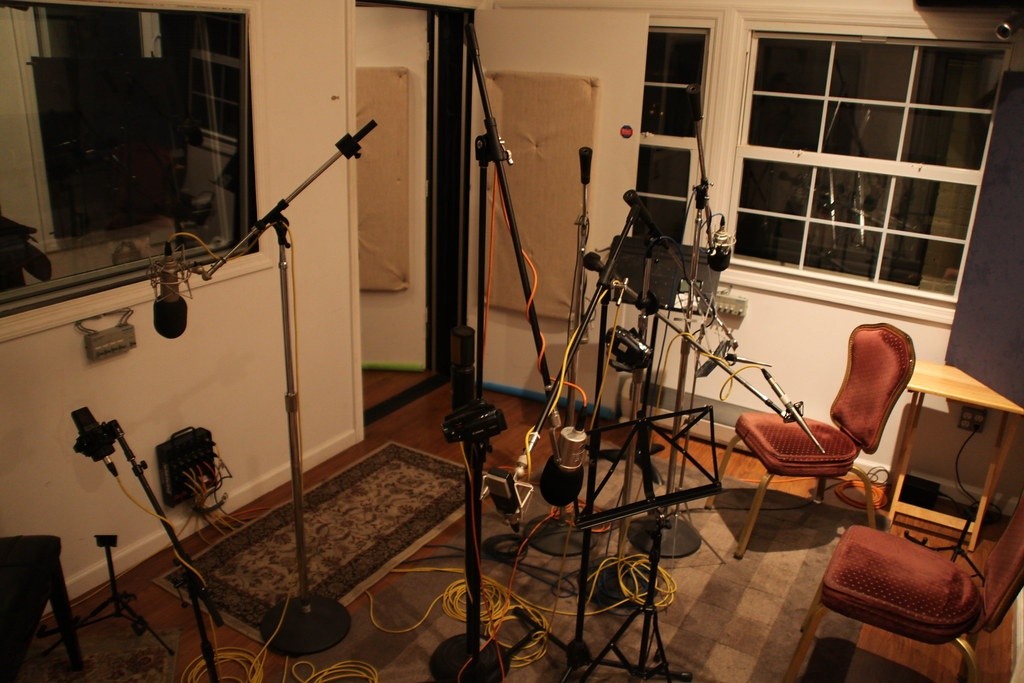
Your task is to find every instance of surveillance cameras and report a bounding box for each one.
[995,12,1024,40]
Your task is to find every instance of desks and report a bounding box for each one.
[885,361,1024,552]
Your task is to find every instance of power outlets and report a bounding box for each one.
[957,405,987,435]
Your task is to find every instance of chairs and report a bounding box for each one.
[783,493,1024,683]
[0,535,85,683]
[707,322,916,561]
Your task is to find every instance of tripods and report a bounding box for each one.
[33,532,175,659]
[511,209,724,682]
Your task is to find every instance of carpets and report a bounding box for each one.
[152,440,490,645]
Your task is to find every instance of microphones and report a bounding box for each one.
[153,259,188,340]
[708,228,732,272]
[581,251,626,291]
[485,466,522,533]
[71,406,118,476]
[539,429,587,510]
